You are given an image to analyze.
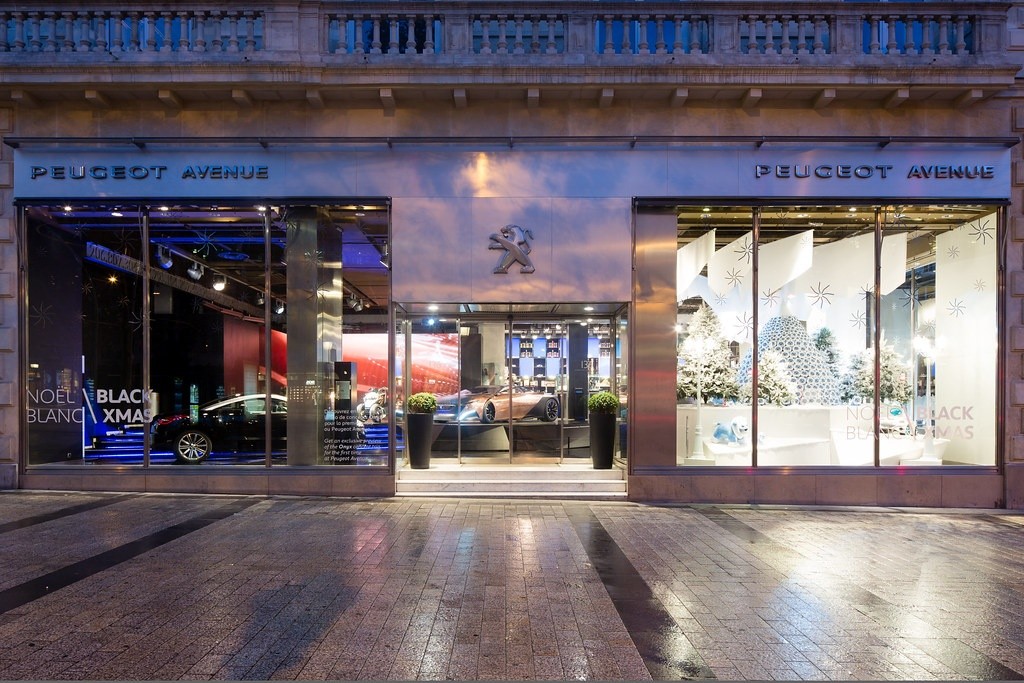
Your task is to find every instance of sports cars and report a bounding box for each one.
[433,385,560,424]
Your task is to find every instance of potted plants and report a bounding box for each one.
[406,393,437,469]
[586,392,619,469]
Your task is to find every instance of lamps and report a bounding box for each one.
[111,207,123,216]
[187,262,205,280]
[257,292,264,305]
[379,255,388,267]
[273,300,284,314]
[157,246,172,268]
[212,273,227,291]
[354,299,363,312]
[354,207,365,216]
[346,294,357,308]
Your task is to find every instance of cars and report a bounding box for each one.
[151,394,288,464]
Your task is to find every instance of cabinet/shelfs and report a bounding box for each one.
[533,358,546,380]
[520,339,534,358]
[599,338,611,358]
[508,358,519,379]
[545,339,560,358]
